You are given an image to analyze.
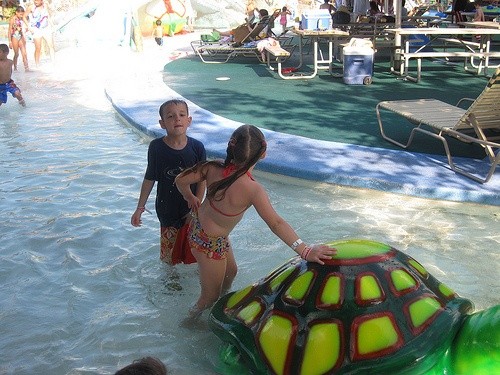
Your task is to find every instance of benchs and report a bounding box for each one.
[400,53,500,83]
[438,37,486,68]
[333,23,418,48]
[265,45,290,78]
[334,40,377,53]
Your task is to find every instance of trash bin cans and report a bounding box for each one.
[342,37,375,85]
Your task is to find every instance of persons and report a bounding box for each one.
[246,0,260,24]
[131,100,207,263]
[473,2,484,43]
[402,0,407,16]
[22,0,54,64]
[213,9,268,36]
[332,5,351,24]
[152,20,163,46]
[8,6,30,70]
[280,6,292,31]
[367,0,394,40]
[320,0,337,14]
[176,124,336,311]
[0,44,22,105]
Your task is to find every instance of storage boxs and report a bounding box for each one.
[343,47,376,86]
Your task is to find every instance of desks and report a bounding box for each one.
[459,11,500,17]
[384,27,500,83]
[393,15,439,40]
[428,19,456,24]
[456,21,500,29]
[288,28,349,80]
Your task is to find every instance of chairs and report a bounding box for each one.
[376,68,500,183]
[191,8,281,63]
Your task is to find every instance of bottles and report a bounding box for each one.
[230,34,234,42]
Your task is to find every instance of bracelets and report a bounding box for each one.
[137,206,145,212]
[291,239,303,251]
[301,246,312,260]
